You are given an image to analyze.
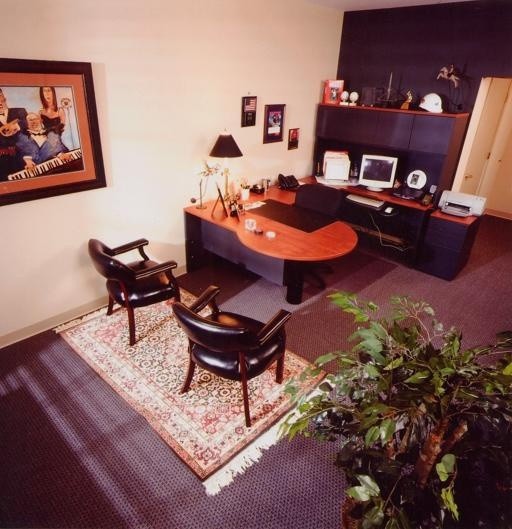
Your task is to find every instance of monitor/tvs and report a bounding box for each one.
[358,154,398,192]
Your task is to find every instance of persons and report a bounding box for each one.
[0,86,72,181]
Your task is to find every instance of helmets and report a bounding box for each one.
[418,93,444,113]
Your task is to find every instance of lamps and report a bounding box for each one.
[210,131,246,195]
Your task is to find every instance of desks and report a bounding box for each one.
[184,175,434,305]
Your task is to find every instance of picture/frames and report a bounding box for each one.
[0,54,109,205]
[286,127,300,153]
[260,103,285,144]
[241,95,257,126]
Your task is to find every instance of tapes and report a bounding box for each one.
[255,229,263,235]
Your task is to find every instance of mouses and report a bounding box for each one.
[384,207,395,215]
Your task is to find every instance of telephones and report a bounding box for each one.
[278,174,299,189]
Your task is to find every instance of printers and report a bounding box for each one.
[438,190,488,218]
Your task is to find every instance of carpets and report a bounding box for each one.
[51,286,337,498]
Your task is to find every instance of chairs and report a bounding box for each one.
[166,285,293,428]
[295,181,346,289]
[88,235,185,346]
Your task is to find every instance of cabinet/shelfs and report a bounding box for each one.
[419,206,480,281]
[316,103,468,157]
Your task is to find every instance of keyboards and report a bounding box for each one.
[345,194,385,207]
